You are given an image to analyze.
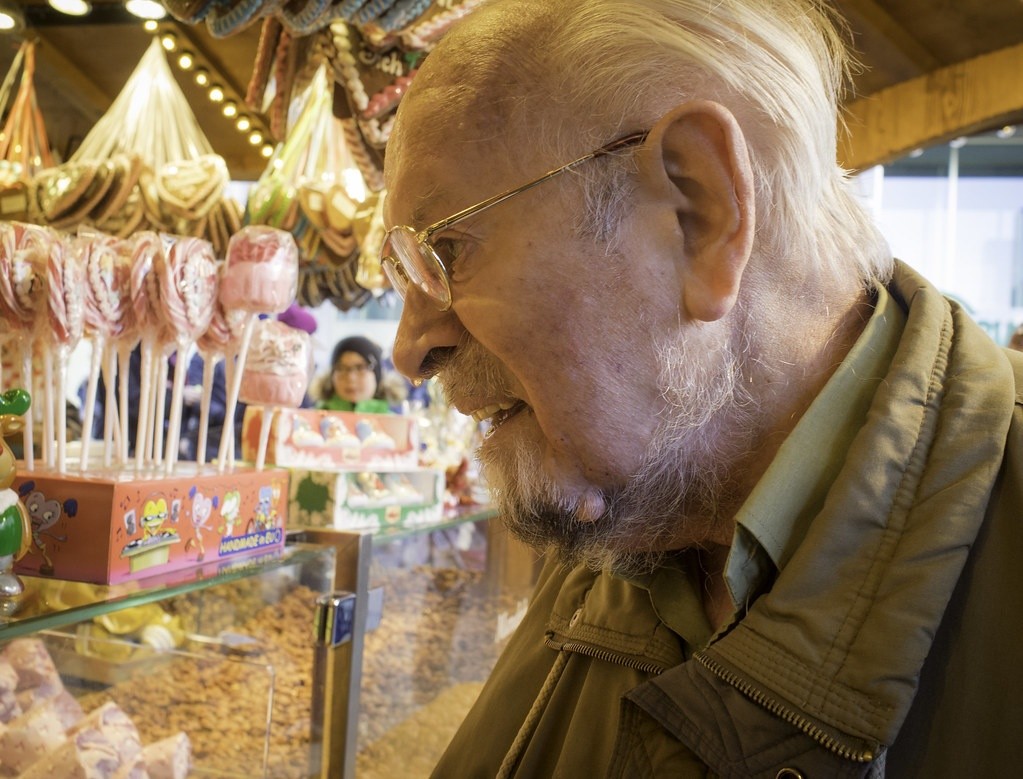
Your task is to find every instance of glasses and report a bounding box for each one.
[333,364,373,375]
[381,131,647,312]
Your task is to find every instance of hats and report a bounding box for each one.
[260,305,317,334]
[332,336,384,388]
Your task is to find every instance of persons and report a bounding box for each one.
[383,0,1022,779]
[308,336,402,418]
[77,302,321,468]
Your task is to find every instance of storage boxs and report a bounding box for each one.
[12,461,292,586]
[286,468,446,533]
[241,405,410,468]
